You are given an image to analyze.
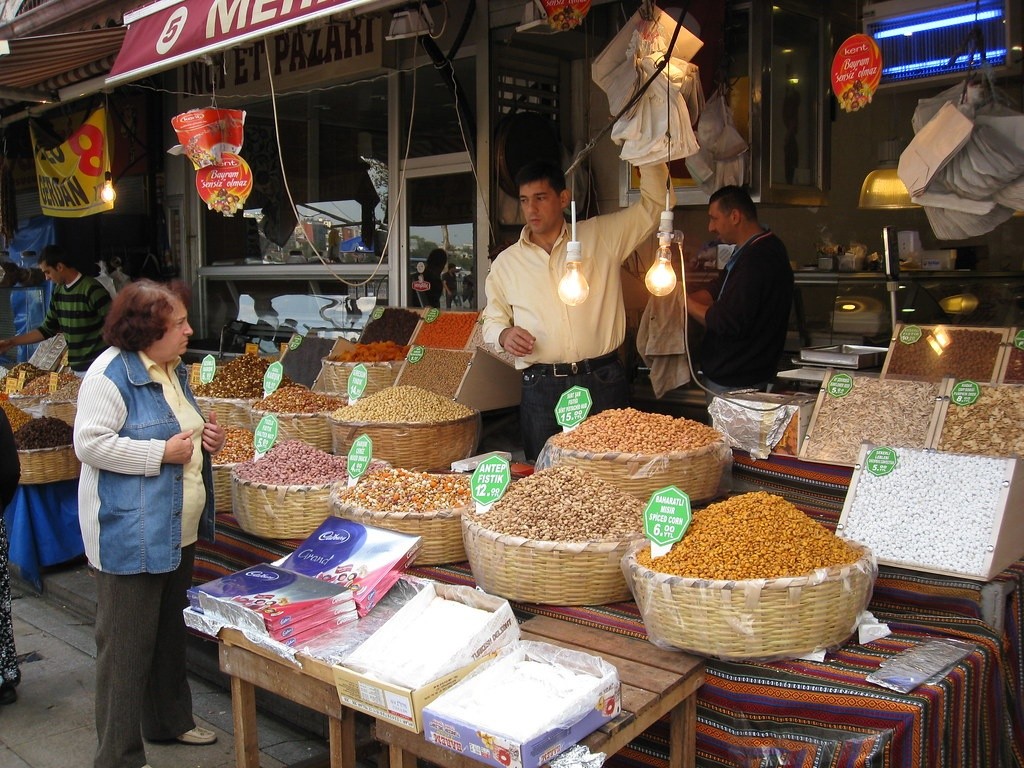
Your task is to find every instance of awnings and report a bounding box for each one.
[103,0,404,92]
[0,24,130,104]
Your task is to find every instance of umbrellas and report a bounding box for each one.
[339,236,378,264]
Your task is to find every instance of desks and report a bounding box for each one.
[182,517,706,768]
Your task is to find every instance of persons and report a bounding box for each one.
[0,405,25,701]
[0,245,115,381]
[67,280,230,768]
[685,178,797,424]
[409,248,475,311]
[479,157,676,467]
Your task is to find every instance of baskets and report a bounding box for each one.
[192,359,875,659]
[9,395,83,485]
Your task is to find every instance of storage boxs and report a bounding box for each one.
[423,638,620,768]
[333,581,521,735]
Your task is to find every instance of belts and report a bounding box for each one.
[528,348,620,377]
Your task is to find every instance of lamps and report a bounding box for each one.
[859,138,925,211]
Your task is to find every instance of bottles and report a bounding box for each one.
[285,250,328,264]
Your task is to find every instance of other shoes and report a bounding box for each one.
[0,690,18,705]
[176,724,216,745]
